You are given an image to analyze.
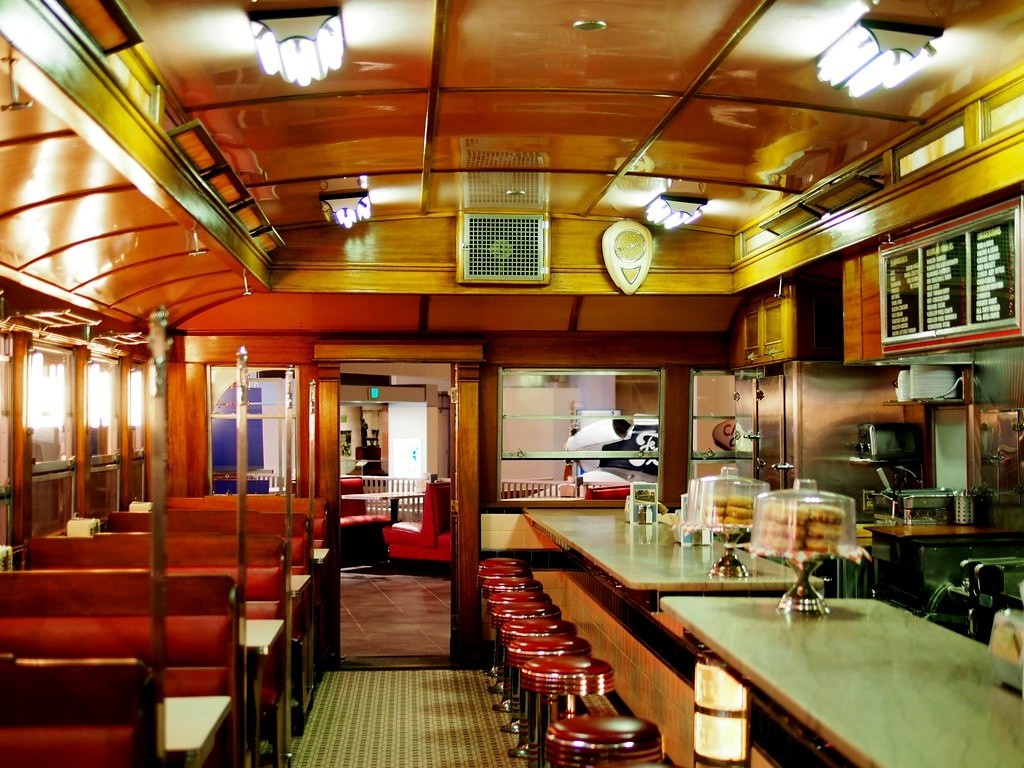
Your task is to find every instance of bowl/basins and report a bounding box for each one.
[897,370,912,402]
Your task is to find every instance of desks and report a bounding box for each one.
[291,575,311,597]
[525,508,1024,768]
[314,549,329,564]
[163,696,231,768]
[341,491,425,571]
[246,619,285,654]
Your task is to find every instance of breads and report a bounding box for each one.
[697,496,754,525]
[764,503,845,551]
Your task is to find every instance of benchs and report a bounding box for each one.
[0,498,330,768]
[340,476,400,567]
[383,482,452,574]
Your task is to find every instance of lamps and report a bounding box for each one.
[816,17,946,97]
[319,190,371,229]
[248,6,344,86]
[646,195,709,230]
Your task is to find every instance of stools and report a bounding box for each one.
[476,557,663,768]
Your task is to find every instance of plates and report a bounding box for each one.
[909,364,958,399]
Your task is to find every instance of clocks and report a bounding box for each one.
[602,219,654,296]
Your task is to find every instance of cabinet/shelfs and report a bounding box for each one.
[729,283,906,523]
